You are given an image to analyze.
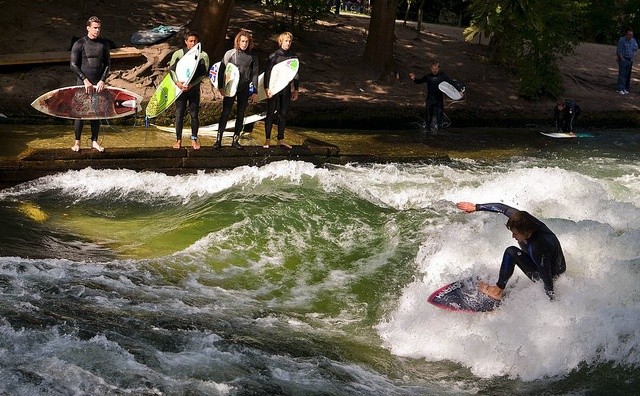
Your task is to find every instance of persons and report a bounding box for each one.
[616,30,639,95]
[263,32,301,150]
[69,13,112,154]
[408,60,468,147]
[213,29,259,152]
[552,98,584,137]
[167,28,206,151]
[453,200,567,302]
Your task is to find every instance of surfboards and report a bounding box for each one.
[427,276,516,314]
[247,58,299,103]
[438,81,464,100]
[150,122,250,137]
[201,110,277,130]
[540,132,601,138]
[31,85,143,119]
[209,62,240,97]
[145,42,201,119]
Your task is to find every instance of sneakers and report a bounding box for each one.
[616,89,629,94]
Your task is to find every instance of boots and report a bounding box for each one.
[213,133,222,149]
[232,135,242,149]
